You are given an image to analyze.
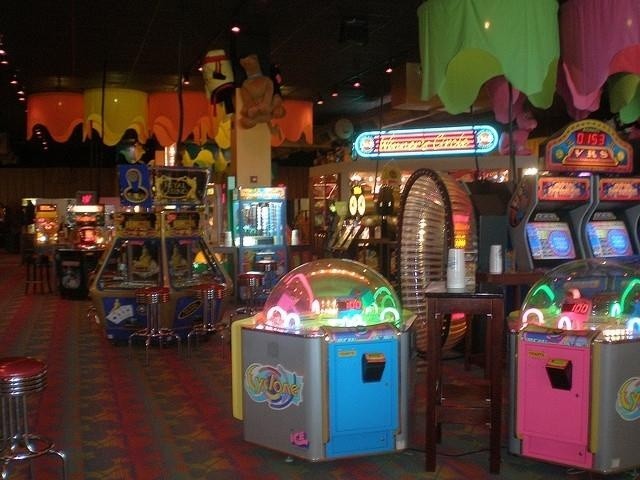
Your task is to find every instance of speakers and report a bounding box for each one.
[391,61,431,112]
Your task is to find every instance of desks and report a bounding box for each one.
[476,266,545,315]
[419,276,513,475]
[290,244,315,266]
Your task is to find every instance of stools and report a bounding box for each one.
[254,258,277,301]
[25,252,54,299]
[2,351,70,478]
[188,282,232,347]
[125,286,183,355]
[235,268,262,319]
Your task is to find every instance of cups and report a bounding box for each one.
[489,245,503,273]
[446,248,466,288]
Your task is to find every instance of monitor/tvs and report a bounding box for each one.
[527,221,577,260]
[587,220,634,259]
